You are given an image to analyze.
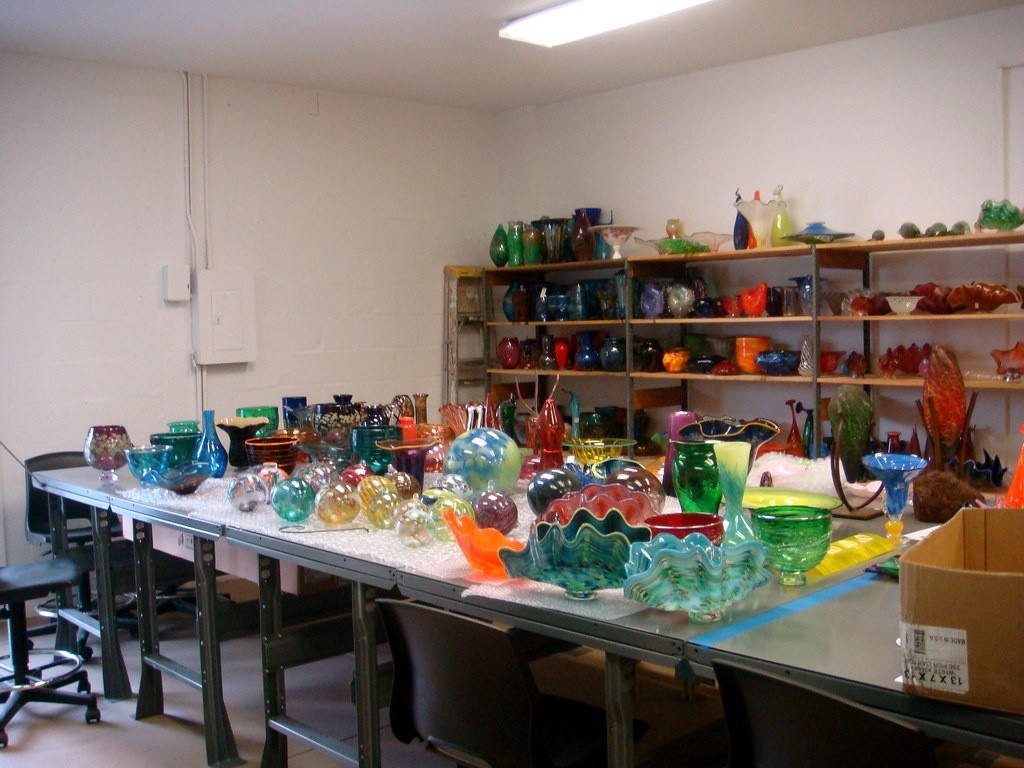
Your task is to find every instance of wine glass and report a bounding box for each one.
[83,426,134,485]
[862,452,930,545]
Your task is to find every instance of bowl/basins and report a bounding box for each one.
[123,445,174,486]
[885,297,924,314]
[150,432,206,465]
[644,512,727,544]
[721,487,848,509]
[562,438,637,464]
[148,460,219,494]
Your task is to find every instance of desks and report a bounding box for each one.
[29,465,1024,768]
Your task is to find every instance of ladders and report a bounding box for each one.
[443,265,499,425]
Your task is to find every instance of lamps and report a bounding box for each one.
[499,0,708,48]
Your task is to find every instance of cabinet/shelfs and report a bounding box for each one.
[479,230,1024,484]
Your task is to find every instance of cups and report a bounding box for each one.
[236,406,279,438]
[351,426,402,475]
[751,505,835,584]
[244,437,302,475]
[215,416,271,466]
[283,396,307,429]
[734,337,770,374]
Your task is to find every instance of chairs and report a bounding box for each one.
[373,596,611,768]
[0,557,99,748]
[153,550,236,621]
[25,451,139,659]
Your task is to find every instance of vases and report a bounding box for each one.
[85,182,1024,626]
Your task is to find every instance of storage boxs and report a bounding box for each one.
[898,505,1024,716]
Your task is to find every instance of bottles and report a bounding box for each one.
[166,420,200,433]
[399,416,416,441]
[191,410,233,475]
[886,431,902,455]
[490,207,814,377]
[538,398,564,469]
